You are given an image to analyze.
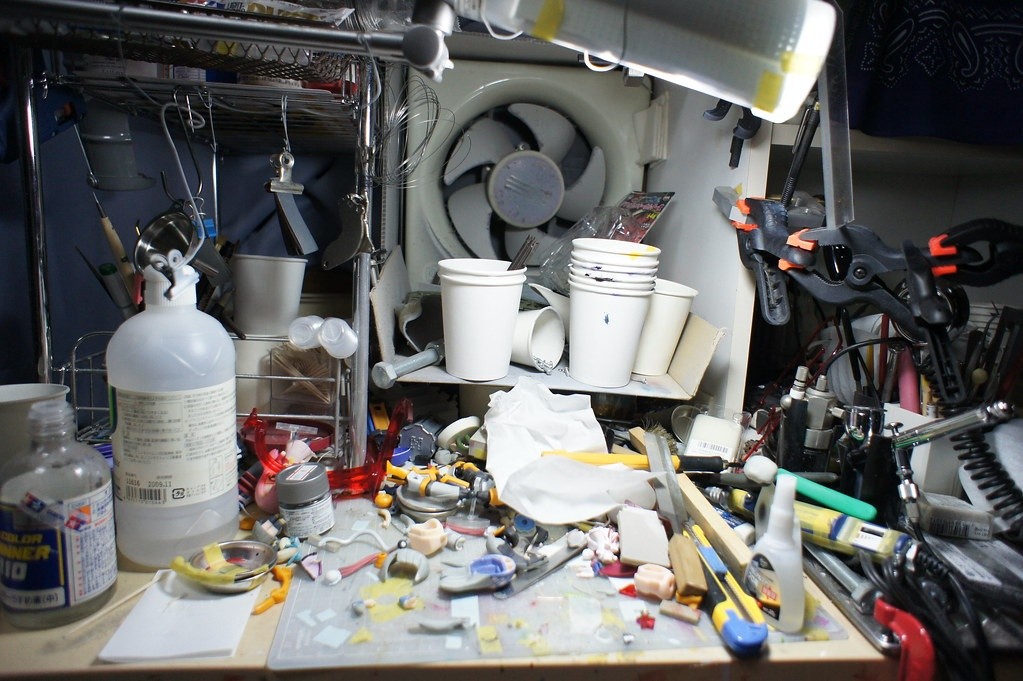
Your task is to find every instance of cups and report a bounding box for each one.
[0,384,70,461]
[567,238,699,388]
[511,306,565,372]
[318,317,358,359]
[231,254,308,336]
[437,258,527,381]
[288,315,324,349]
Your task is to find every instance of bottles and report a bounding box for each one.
[0,400,118,628]
[745,475,805,633]
[106,264,239,567]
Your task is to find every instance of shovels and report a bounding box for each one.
[642,432,709,598]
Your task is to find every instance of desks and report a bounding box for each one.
[0,489,1023,681]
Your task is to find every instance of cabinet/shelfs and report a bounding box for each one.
[30,1,384,497]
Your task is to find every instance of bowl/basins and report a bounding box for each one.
[671,405,702,444]
[189,540,278,593]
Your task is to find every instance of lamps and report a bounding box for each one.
[0,0,839,125]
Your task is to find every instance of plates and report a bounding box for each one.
[396,484,461,522]
